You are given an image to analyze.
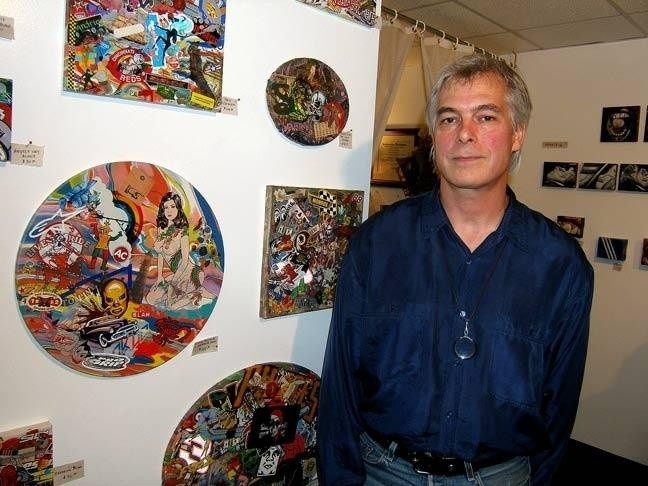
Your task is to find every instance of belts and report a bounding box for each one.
[372,430,515,476]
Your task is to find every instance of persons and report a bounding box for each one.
[312,54,595,486]
